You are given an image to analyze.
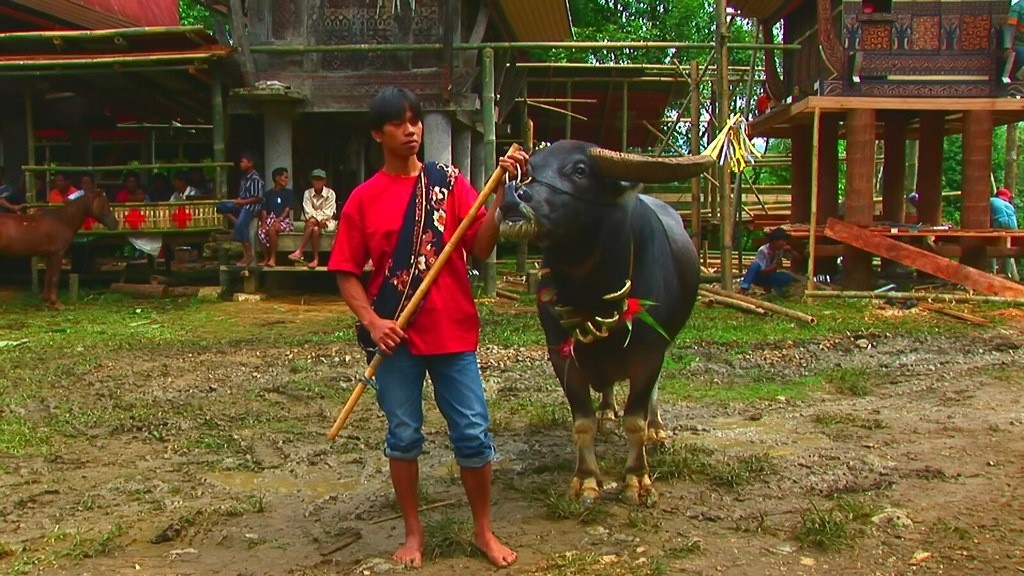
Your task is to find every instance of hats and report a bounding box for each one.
[995,188,1010,202]
[909,193,918,205]
[765,227,790,239]
[312,169,326,178]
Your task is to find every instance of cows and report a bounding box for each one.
[490,113,764,515]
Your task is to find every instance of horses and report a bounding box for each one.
[0,176,123,310]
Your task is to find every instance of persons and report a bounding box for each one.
[259,167,295,267]
[738,227,800,294]
[327,85,529,569]
[990,188,1020,282]
[216,150,264,267]
[288,168,336,269]
[905,188,919,224]
[0,162,215,280]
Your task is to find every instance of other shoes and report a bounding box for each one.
[288,254,304,263]
[307,261,317,269]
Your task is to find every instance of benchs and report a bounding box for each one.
[255,221,336,252]
